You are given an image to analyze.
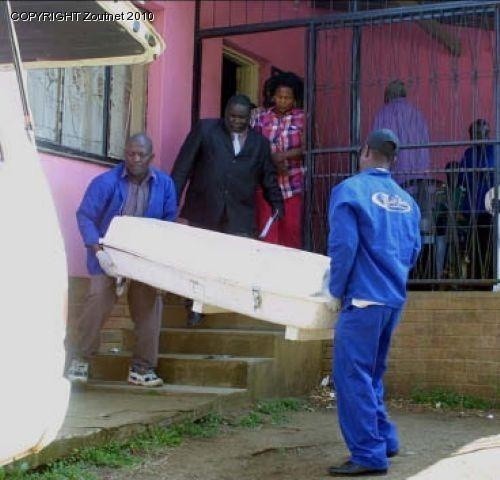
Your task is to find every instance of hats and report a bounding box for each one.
[231,133,242,155]
[365,128,399,157]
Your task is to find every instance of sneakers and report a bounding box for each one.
[126,362,163,388]
[66,357,89,387]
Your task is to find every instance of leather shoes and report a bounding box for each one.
[329,459,388,477]
[186,305,204,327]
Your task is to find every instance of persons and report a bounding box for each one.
[372,79,433,204]
[250,71,306,249]
[170,94,287,328]
[326,127,422,477]
[433,161,466,237]
[460,119,495,278]
[66,133,178,387]
